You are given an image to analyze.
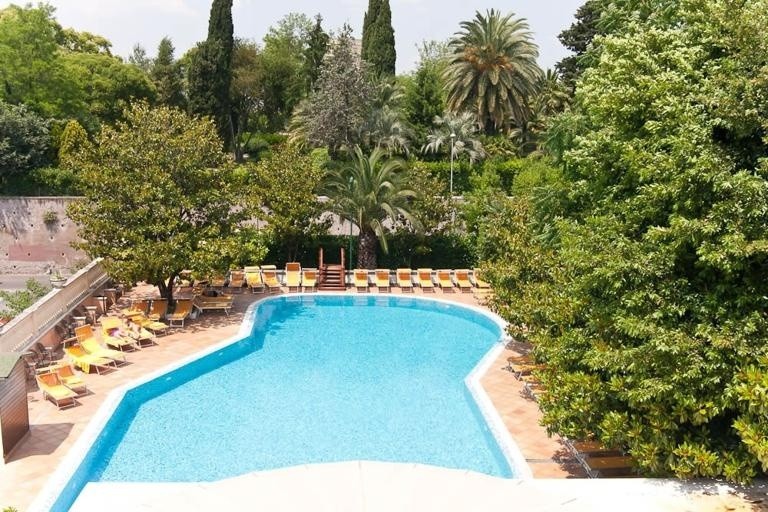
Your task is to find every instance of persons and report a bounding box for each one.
[122,313,141,334]
[107,327,129,342]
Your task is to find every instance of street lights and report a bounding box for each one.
[450,131,457,197]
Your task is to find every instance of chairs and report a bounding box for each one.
[355,268,492,295]
[210,262,317,296]
[18,286,237,412]
